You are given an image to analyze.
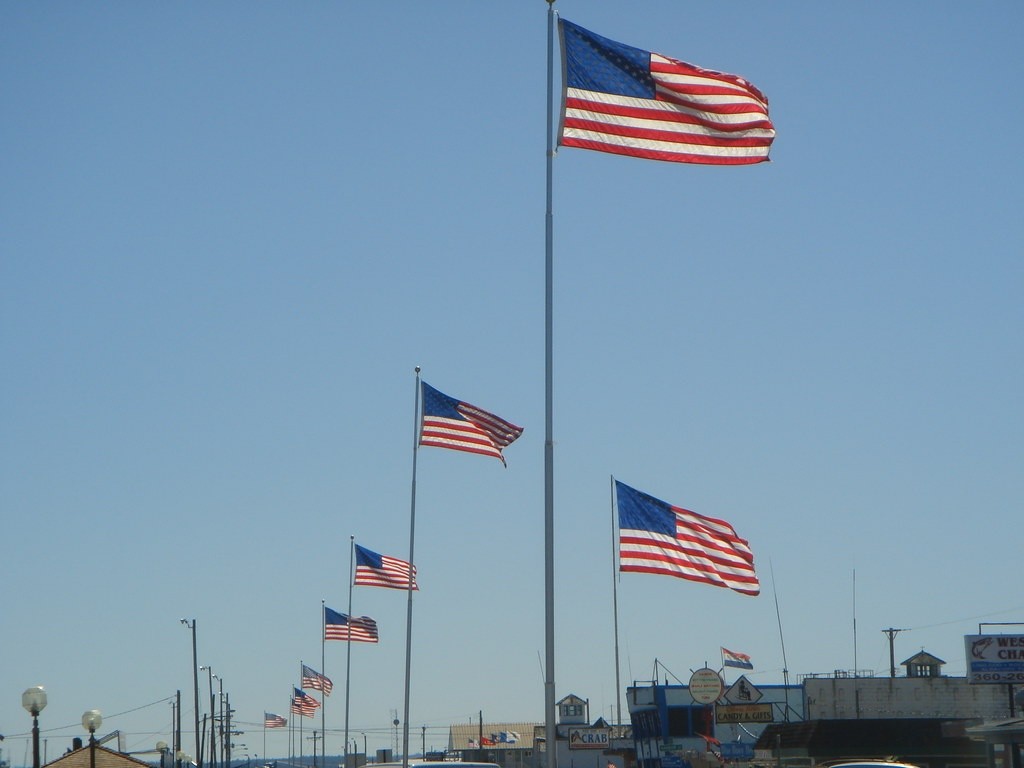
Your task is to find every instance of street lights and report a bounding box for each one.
[156,742,166,768]
[180,618,201,768]
[185,755,192,768]
[200,665,217,768]
[81,709,102,768]
[22,685,48,768]
[176,751,184,768]
[211,673,249,768]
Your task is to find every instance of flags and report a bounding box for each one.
[555,18,775,163]
[325,607,378,643]
[355,546,420,590]
[469,731,520,747]
[419,383,523,466]
[292,700,315,718]
[723,649,754,669]
[303,666,332,696]
[295,689,321,708]
[616,481,761,596]
[265,714,287,728]
